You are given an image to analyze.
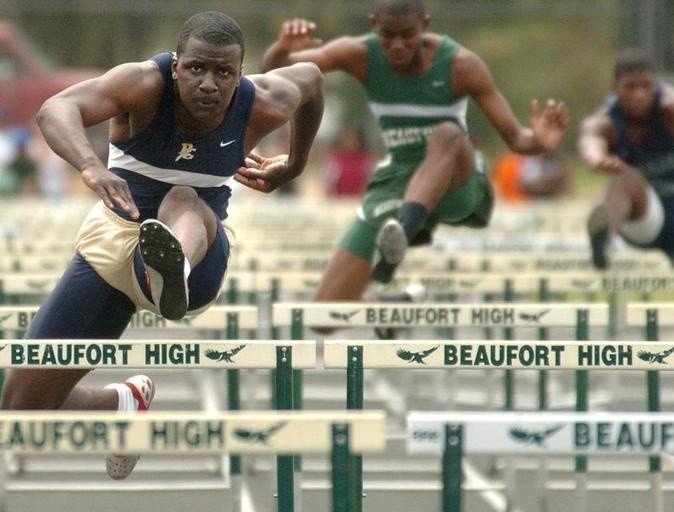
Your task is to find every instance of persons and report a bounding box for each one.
[258,118,378,198]
[256,3,575,340]
[1,111,108,205]
[491,150,566,202]
[0,8,327,487]
[573,44,672,275]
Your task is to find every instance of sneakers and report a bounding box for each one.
[367,218,407,284]
[137,219,189,321]
[587,205,612,270]
[105,374,155,479]
[374,283,427,340]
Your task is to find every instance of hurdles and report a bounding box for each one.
[0,252,386,512]
[245,252,672,512]
